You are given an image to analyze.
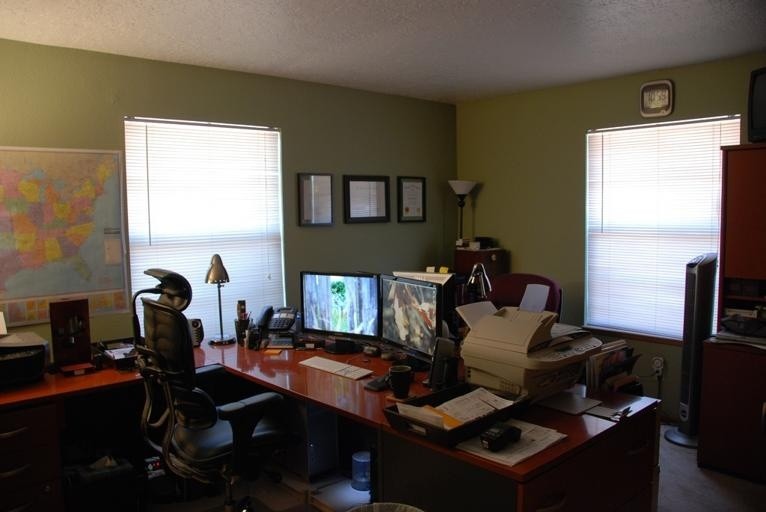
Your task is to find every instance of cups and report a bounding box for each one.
[389,365,411,400]
[234,320,249,345]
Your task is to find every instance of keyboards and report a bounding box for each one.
[299,356,373,380]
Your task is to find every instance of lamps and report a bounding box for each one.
[205,254,237,348]
[448,179,477,248]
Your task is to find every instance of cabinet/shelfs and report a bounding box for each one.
[717,143,766,340]
[456,250,508,283]
[698,338,766,488]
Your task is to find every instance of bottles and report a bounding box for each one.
[252,327,261,351]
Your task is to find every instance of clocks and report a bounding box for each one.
[639,80,674,116]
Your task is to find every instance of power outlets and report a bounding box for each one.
[651,356,664,377]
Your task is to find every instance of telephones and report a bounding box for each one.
[258,306,298,330]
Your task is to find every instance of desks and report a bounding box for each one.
[0,332,664,507]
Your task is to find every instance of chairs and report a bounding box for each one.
[131,269,286,511]
[486,272,562,323]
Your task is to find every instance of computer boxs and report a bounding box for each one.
[289,398,340,482]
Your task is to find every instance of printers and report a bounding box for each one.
[0,311,50,389]
[460,284,603,401]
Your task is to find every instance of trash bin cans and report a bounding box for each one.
[346,502,424,511]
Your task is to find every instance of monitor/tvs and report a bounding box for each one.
[300,271,381,354]
[380,273,442,371]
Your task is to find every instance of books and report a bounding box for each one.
[586,340,643,394]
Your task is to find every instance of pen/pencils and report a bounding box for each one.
[247,312,251,320]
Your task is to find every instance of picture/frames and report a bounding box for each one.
[342,173,390,224]
[296,173,334,227]
[396,176,427,222]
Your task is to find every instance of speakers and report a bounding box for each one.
[429,337,455,392]
